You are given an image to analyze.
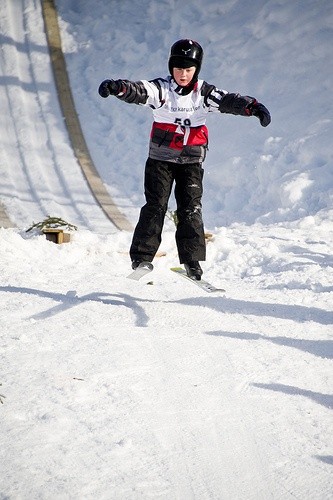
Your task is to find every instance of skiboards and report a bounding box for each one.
[124,260,226,295]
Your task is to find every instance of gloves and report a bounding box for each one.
[98,79,122,98]
[250,102,270,128]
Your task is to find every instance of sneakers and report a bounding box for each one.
[131,259,143,270]
[183,261,203,281]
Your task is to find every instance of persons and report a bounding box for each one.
[98,39,271,279]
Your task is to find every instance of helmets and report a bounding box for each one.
[171,39,203,61]
